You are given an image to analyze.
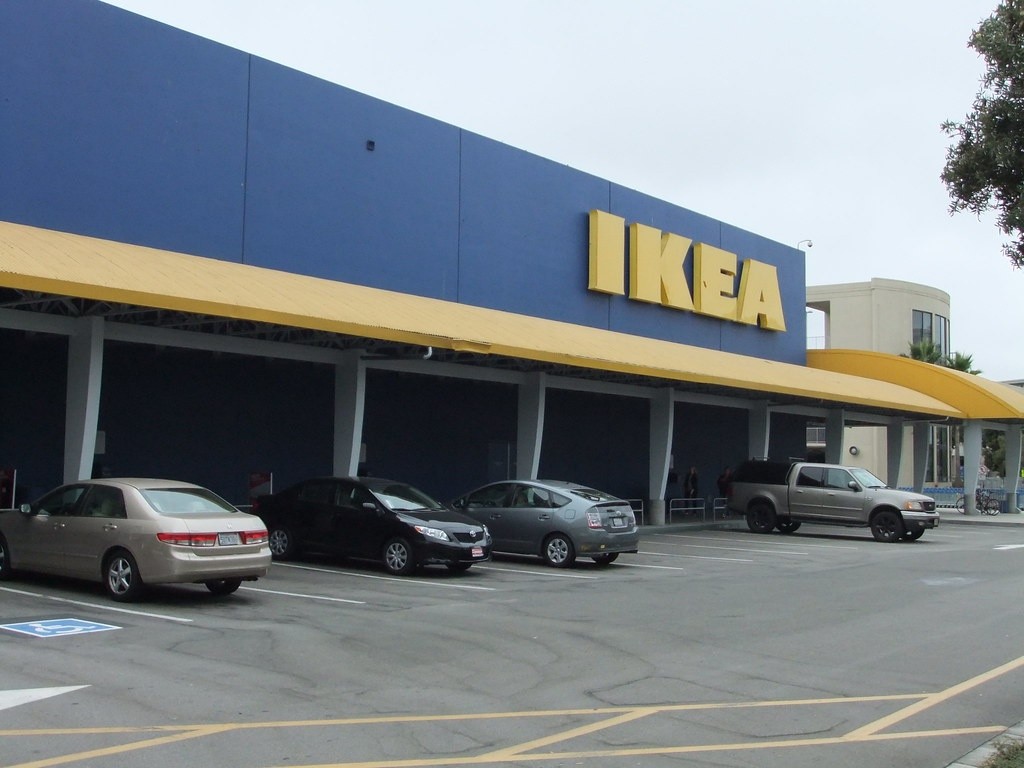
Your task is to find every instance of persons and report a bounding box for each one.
[681,465,699,517]
[715,466,735,519]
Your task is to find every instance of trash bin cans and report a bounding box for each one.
[1007,492,1018,514]
[999,500,1007,513]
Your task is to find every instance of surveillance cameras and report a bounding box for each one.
[808,241,812,247]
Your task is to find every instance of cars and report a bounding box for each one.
[1,473,271,607]
[441,474,646,570]
[251,473,494,581]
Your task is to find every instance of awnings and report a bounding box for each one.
[0,222,968,425]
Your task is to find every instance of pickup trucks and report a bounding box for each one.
[722,459,939,545]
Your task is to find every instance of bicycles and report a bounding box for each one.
[954,489,1003,519]
